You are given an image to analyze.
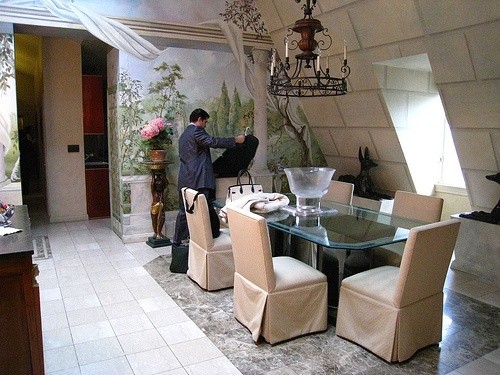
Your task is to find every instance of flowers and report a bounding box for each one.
[138,117,174,150]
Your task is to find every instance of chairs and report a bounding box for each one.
[182,176,461,366]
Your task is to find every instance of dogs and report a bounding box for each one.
[459,172,500,225]
[337,147,393,201]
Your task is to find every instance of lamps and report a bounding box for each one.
[267,0,351,98]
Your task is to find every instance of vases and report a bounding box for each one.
[150,148,167,162]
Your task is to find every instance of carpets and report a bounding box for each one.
[32,235,52,260]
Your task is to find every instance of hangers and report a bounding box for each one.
[244,126,251,136]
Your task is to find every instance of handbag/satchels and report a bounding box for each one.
[170,241,189,274]
[206,200,220,239]
[226,169,263,205]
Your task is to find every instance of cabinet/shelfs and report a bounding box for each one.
[85,168,111,220]
[81,73,107,136]
[0,206,45,375]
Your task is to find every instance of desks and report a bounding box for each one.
[212,193,432,328]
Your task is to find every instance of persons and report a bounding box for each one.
[171,108,245,255]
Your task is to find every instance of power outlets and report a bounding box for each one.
[68,145,79,152]
